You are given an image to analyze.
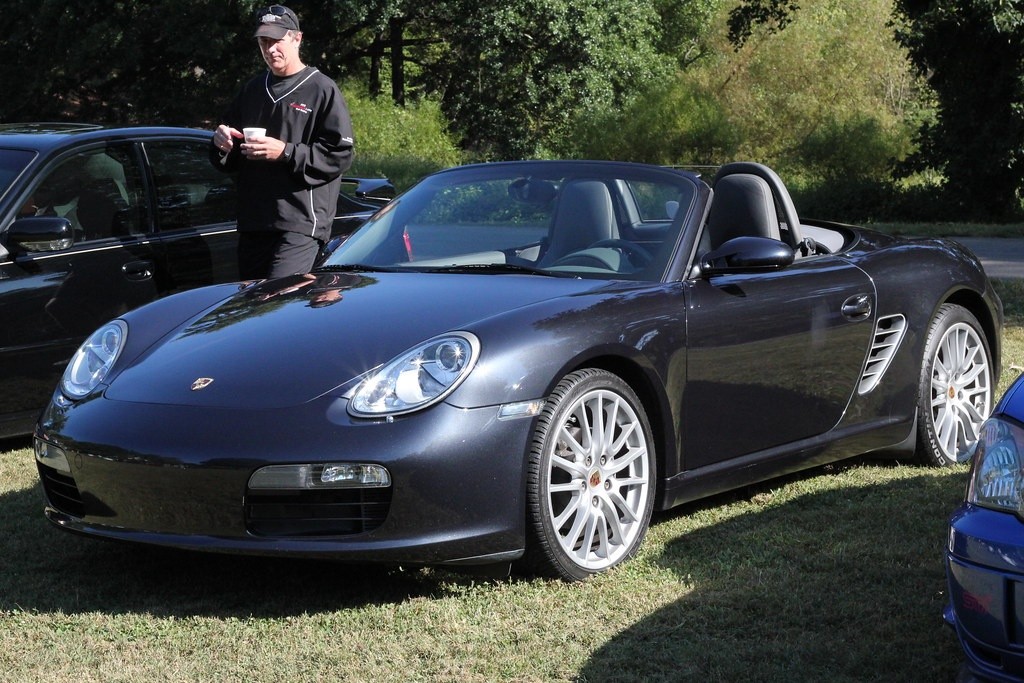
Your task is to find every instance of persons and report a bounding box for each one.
[261,272,361,308]
[208,4,354,282]
[77,153,130,241]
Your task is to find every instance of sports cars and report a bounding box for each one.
[33,160,1005,580]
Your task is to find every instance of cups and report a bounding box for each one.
[243,128,267,149]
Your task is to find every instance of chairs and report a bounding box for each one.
[537,180,623,268]
[76,180,132,240]
[708,174,782,253]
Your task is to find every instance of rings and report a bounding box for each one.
[220,145,223,149]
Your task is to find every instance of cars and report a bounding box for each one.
[0,122,395,439]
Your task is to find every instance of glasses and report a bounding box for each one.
[258,7,298,31]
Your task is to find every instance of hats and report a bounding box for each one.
[253,4,300,39]
